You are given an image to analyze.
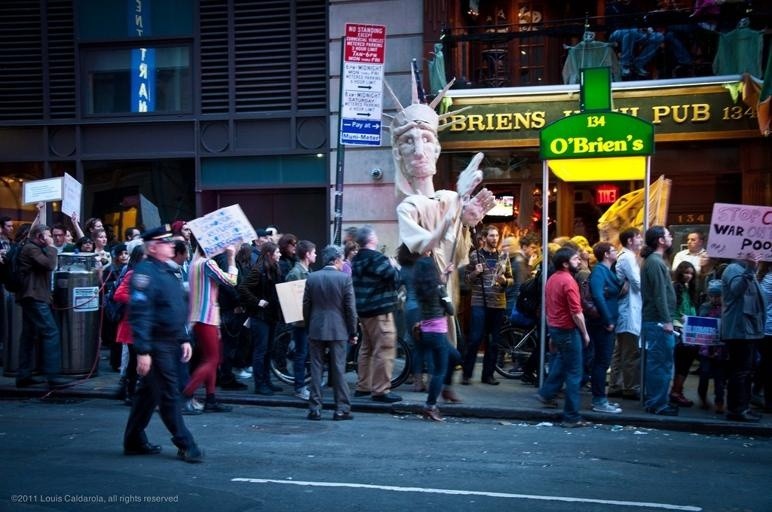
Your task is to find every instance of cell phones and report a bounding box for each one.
[38,234,44,241]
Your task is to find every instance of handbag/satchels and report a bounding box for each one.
[516,278,538,318]
[104,265,127,323]
[509,303,535,329]
[581,280,607,319]
[412,322,421,342]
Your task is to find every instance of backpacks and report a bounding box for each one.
[4,242,36,294]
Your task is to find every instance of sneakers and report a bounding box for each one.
[522,372,763,428]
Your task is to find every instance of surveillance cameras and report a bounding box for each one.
[370,168,383,180]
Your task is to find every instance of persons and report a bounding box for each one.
[603,0,662,76]
[117,221,206,461]
[664,1,722,78]
[1,216,772,429]
[387,104,499,287]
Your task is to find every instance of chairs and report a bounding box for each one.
[615,4,738,79]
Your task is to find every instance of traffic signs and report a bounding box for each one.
[342,60,384,92]
[341,23,386,63]
[339,118,383,146]
[342,90,383,119]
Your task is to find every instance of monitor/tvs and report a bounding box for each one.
[473,192,514,220]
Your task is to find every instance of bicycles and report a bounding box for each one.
[490,312,610,393]
[269,312,412,398]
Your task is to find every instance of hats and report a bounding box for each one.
[256,228,273,236]
[320,244,342,269]
[171,220,186,232]
[708,279,722,297]
[141,224,174,242]
